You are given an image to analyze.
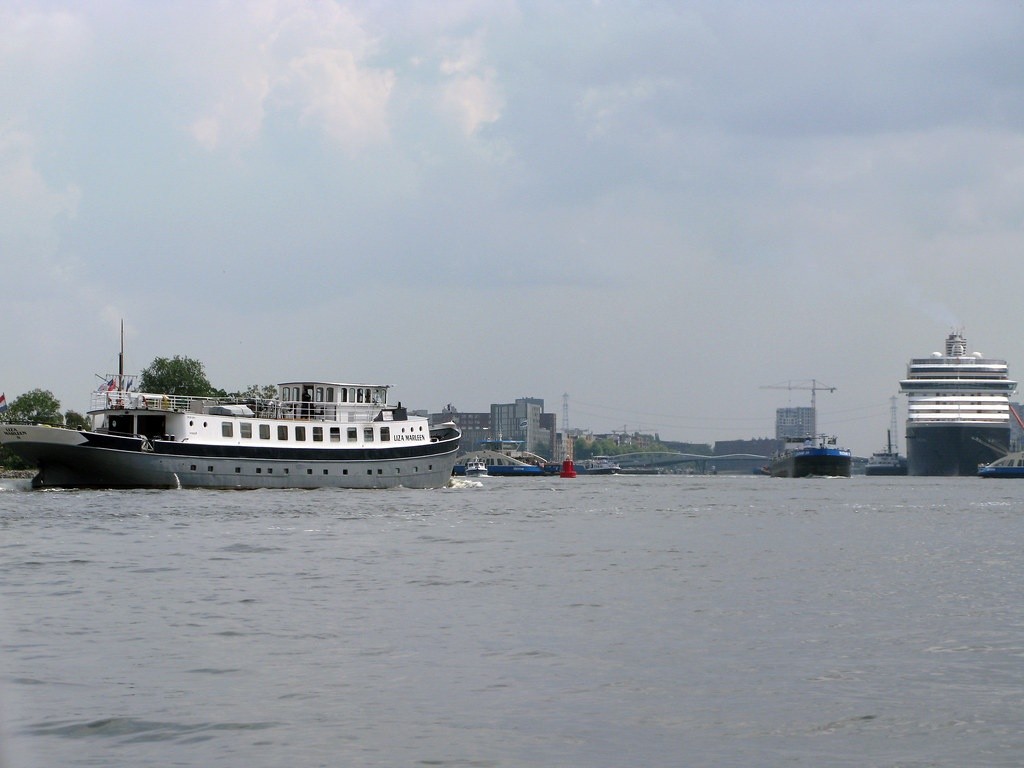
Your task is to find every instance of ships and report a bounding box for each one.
[898,327,1021,476]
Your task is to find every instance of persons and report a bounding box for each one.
[301,388,311,420]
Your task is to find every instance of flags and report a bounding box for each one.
[519,420,527,428]
[0,395,8,413]
[97,378,132,395]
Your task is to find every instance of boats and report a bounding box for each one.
[465,454,490,478]
[769,433,853,479]
[978,452,1024,477]
[864,429,907,476]
[0,317,463,490]
[585,451,621,474]
[453,439,561,477]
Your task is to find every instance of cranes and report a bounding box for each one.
[759,377,836,410]
